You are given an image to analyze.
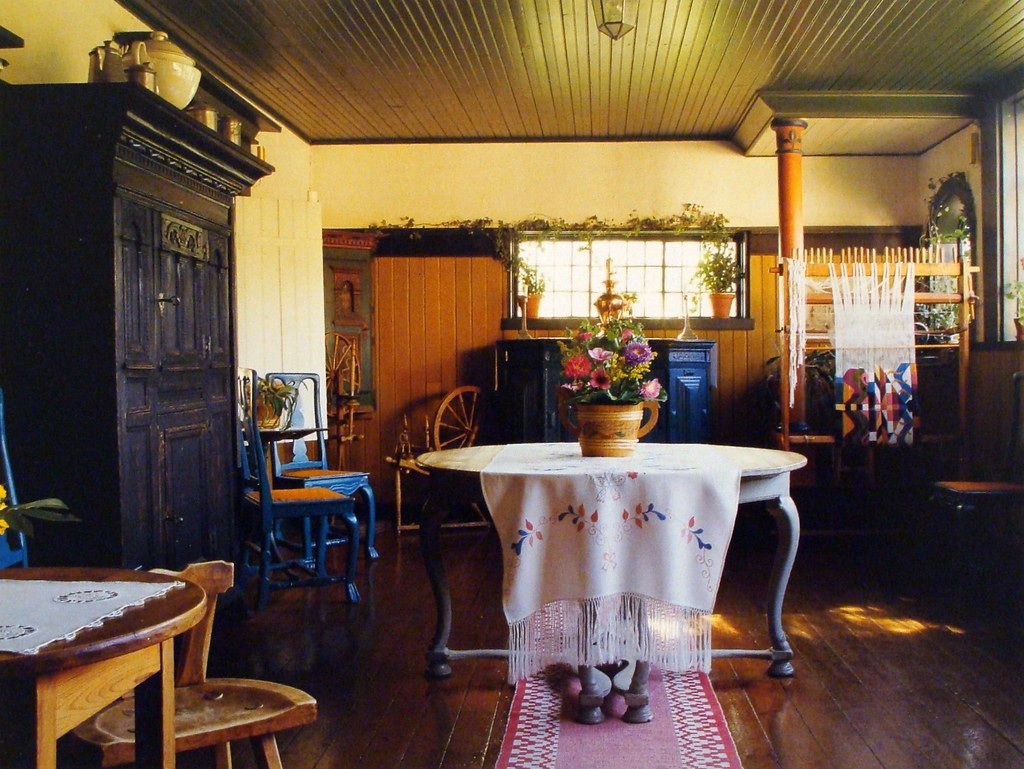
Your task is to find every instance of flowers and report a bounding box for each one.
[558,317,668,407]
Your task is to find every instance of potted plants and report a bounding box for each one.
[517,258,546,319]
[690,226,743,319]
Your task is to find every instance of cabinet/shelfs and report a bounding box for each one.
[1,81,273,616]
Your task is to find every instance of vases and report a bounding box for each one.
[558,397,658,457]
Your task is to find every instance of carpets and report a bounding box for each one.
[495,595,743,769]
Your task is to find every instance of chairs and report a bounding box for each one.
[240,370,378,619]
[72,560,317,769]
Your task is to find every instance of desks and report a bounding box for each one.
[416,443,807,680]
[0,567,207,769]
[493,338,717,448]
